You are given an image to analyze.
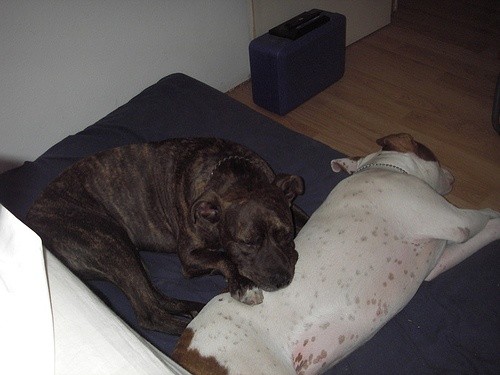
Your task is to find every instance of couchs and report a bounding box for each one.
[0,73,500,375]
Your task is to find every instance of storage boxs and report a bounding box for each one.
[248,9,346,116]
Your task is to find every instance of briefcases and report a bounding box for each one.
[249,9,347,116]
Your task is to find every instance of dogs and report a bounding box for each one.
[170,133,500,375]
[23,137,309,336]
